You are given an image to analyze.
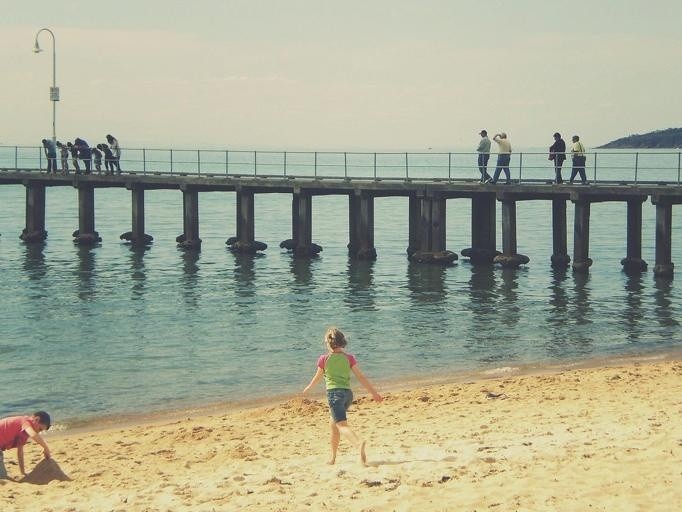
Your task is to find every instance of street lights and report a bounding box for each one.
[32,27,58,151]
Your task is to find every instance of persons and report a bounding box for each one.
[0,411,50,478]
[548,133,567,185]
[566,136,588,184]
[476,130,492,185]
[42,134,122,176]
[489,132,512,184]
[302,327,383,464]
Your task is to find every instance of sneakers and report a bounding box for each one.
[477,177,492,184]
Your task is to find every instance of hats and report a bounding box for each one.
[478,130,487,134]
